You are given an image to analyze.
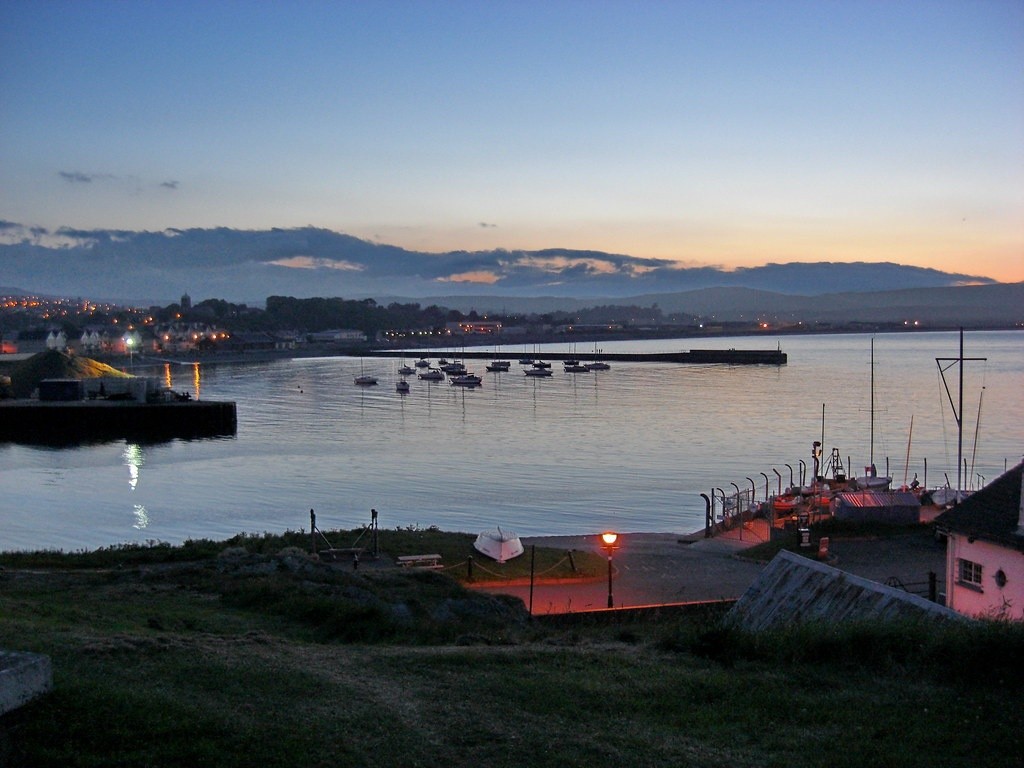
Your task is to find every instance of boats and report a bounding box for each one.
[473,525,525,563]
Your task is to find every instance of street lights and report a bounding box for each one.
[599,530,621,609]
[126,337,134,376]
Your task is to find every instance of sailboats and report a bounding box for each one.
[352,357,379,384]
[853,336,893,492]
[931,326,990,509]
[394,329,611,391]
